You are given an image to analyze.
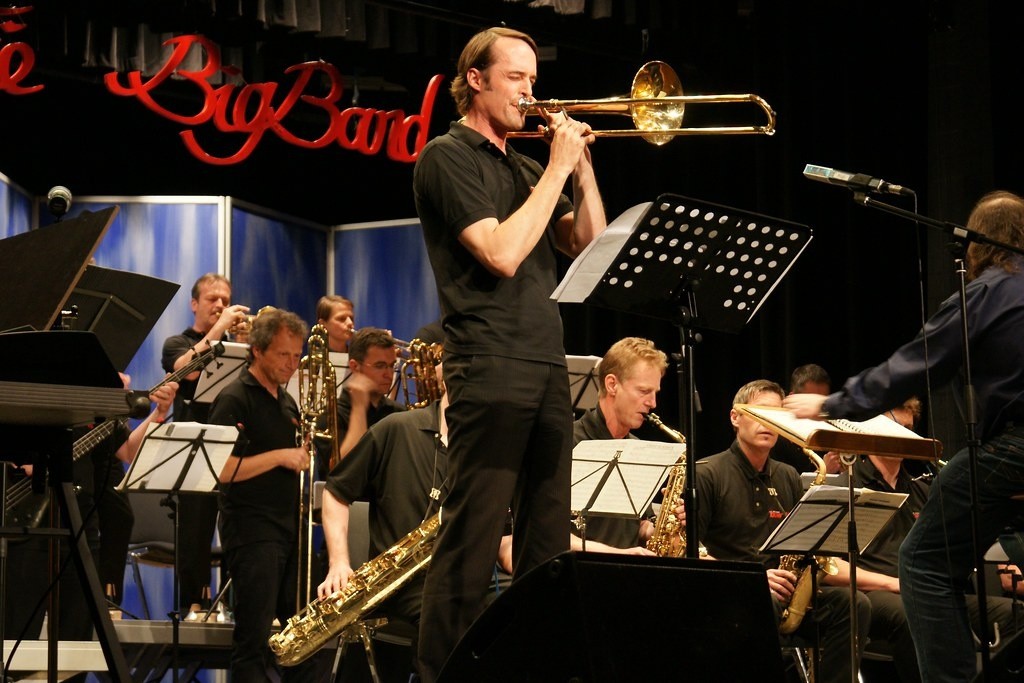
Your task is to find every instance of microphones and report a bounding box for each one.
[47,185,72,223]
[802,163,916,196]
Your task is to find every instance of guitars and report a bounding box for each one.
[0,333,227,534]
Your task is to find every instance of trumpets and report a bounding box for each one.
[214,303,279,346]
[345,326,424,364]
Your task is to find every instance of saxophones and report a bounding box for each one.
[773,445,838,634]
[644,410,691,558]
[268,497,451,672]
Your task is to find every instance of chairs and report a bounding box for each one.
[313,481,417,683]
[127,492,225,619]
[782,635,869,683]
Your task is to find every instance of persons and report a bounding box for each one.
[207,308,312,681]
[569,338,1023,683]
[787,197,1024,683]
[0,372,179,683]
[316,295,356,352]
[315,386,513,632]
[161,272,255,425]
[412,26,607,681]
[317,328,409,482]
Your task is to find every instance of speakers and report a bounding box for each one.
[437,550,790,683]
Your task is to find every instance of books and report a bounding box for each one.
[741,406,923,443]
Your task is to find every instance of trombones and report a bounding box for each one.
[506,59,780,146]
[295,323,448,623]
